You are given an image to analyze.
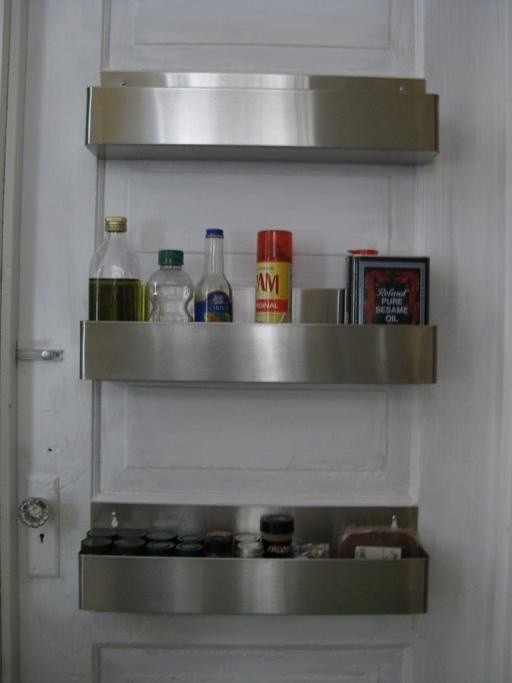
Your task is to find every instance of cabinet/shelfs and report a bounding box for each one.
[76,67,440,620]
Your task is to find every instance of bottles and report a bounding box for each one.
[255,229,293,323]
[147,249,195,322]
[194,227,234,322]
[259,514,294,557]
[88,215,147,321]
